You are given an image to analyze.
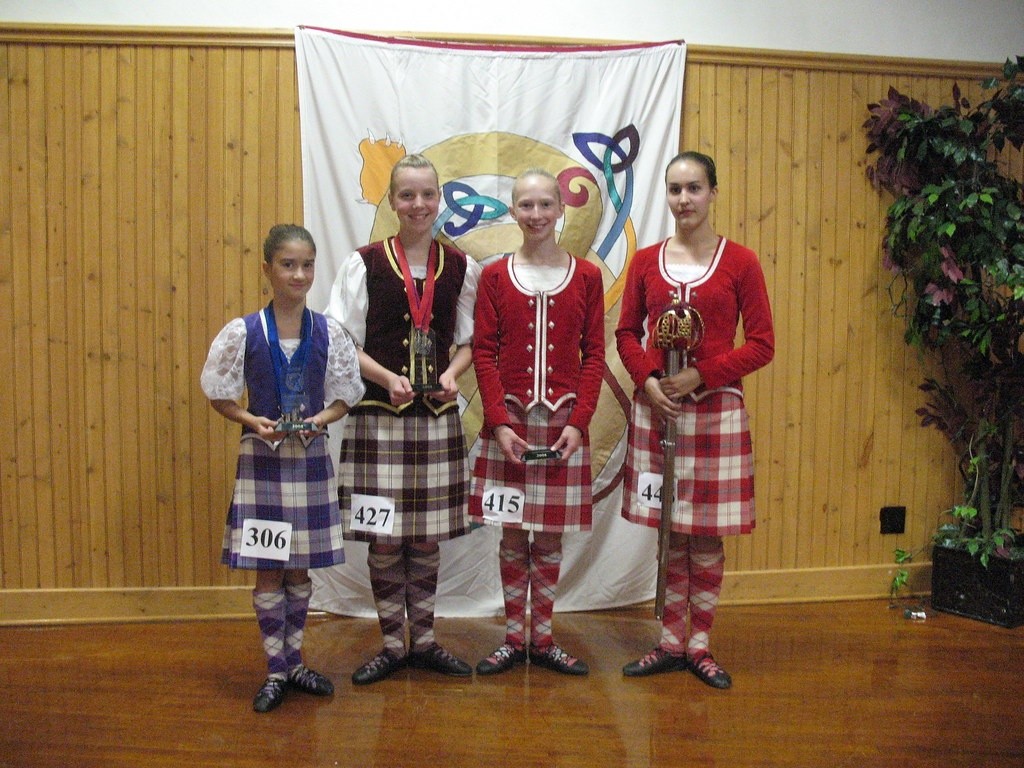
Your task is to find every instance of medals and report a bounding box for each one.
[413,336,433,354]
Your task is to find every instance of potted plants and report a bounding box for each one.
[863,55,1024,629]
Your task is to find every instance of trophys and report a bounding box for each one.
[522,394,556,460]
[406,326,444,393]
[274,361,320,432]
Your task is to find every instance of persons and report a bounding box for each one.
[471,171,605,673]
[615,152,774,690]
[324,153,482,687]
[201,223,364,714]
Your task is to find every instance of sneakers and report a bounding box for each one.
[622,646,688,676]
[351,647,409,685]
[529,641,590,674]
[476,640,528,675]
[286,662,335,695]
[252,673,288,712]
[407,641,474,676]
[684,648,733,688]
